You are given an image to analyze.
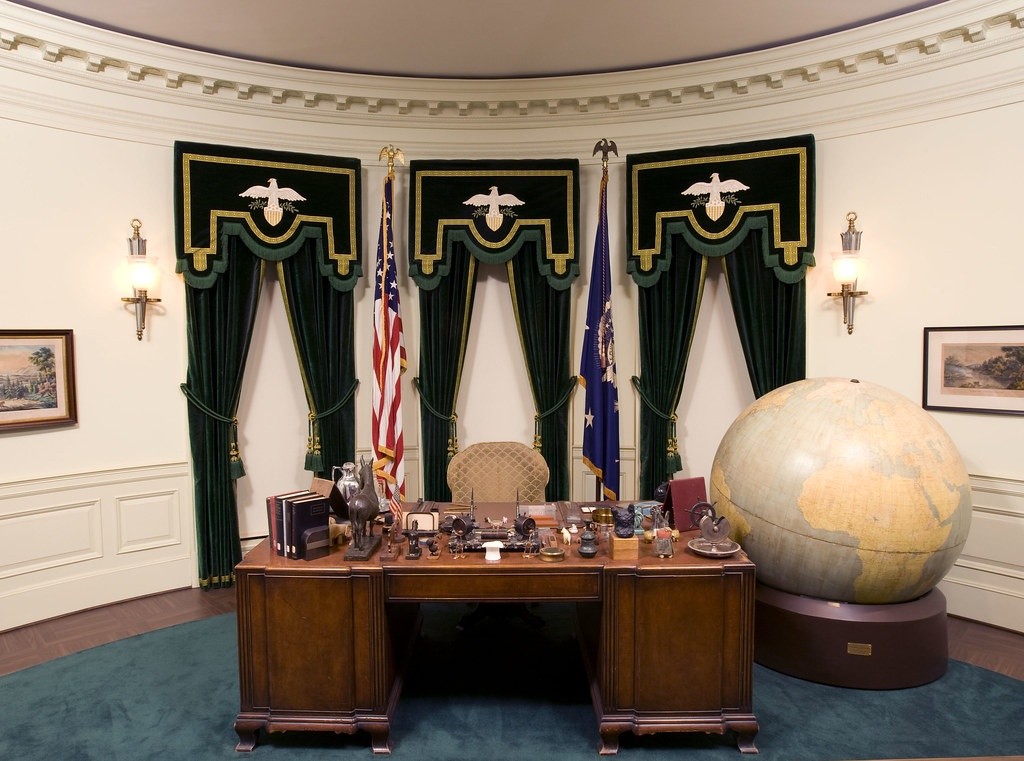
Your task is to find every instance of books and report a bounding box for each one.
[265,488,331,560]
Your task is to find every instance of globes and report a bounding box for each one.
[711,377,975,690]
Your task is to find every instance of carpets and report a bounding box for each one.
[0,597,1024,761]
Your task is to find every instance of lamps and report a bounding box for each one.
[122,218,162,341]
[828,211,868,334]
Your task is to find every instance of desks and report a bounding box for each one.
[229,500,763,755]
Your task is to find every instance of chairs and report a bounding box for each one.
[447,442,545,634]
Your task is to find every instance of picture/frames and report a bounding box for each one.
[922,326,1024,415]
[0,329,79,433]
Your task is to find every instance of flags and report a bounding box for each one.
[577,171,622,502]
[389,480,403,524]
[368,170,409,512]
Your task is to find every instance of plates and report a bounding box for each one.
[688,538,741,557]
